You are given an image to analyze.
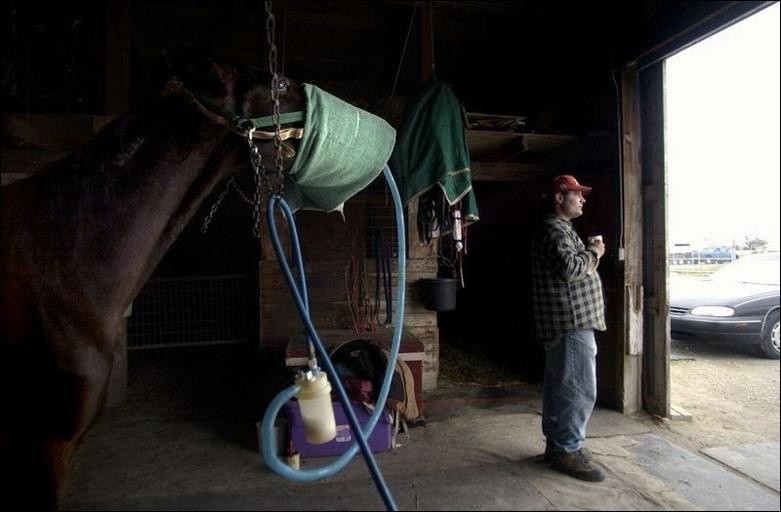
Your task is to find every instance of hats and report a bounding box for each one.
[543,175,592,194]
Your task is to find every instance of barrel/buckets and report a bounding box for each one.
[418,255,459,311]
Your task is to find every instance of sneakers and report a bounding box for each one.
[544,441,605,481]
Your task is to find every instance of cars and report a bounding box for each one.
[670,251,781,360]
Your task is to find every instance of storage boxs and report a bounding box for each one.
[286,326,425,459]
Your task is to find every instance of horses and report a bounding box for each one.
[0,58,307,511]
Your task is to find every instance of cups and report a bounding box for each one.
[587,235,603,244]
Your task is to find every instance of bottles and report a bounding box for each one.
[296,372,337,445]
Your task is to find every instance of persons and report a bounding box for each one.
[531,173,607,482]
